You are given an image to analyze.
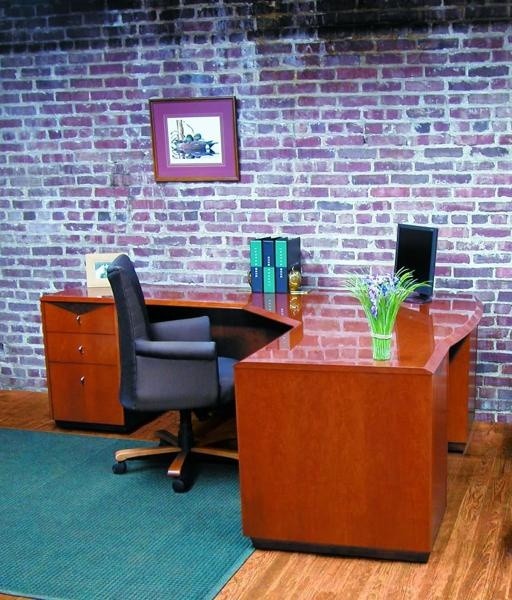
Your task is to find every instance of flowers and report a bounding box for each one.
[334,266,433,359]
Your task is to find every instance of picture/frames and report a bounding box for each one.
[150,97,240,183]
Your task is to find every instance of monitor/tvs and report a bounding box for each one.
[393,223,438,304]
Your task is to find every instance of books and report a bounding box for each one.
[250,236,300,293]
[252,294,303,351]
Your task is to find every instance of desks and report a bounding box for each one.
[40,288,484,565]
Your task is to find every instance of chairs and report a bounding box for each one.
[105,254,236,493]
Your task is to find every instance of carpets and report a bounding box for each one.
[0,427,253,600]
